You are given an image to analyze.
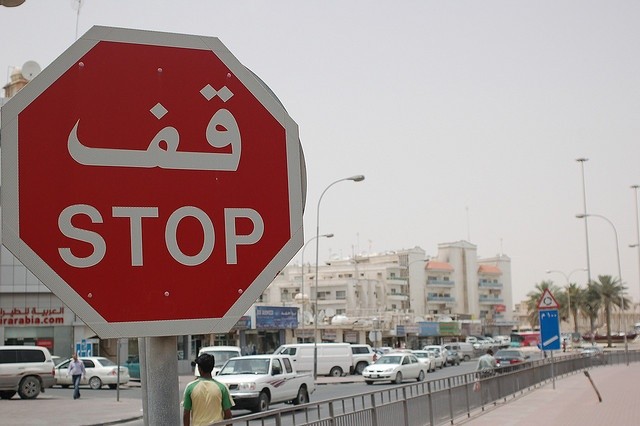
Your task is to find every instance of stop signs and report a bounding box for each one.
[0,26,304,339]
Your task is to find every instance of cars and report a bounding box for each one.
[494,350,530,374]
[55,357,130,390]
[362,353,427,384]
[446,336,510,364]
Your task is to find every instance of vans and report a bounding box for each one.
[0,345,55,399]
[271,343,352,377]
[412,350,436,372]
[191,346,242,376]
[423,345,447,369]
[351,344,376,375]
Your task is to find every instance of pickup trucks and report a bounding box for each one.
[215,354,314,413]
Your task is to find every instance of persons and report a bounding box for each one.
[476,350,496,379]
[183,354,236,426]
[65,355,86,399]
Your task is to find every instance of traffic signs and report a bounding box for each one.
[537,288,559,308]
[540,311,560,350]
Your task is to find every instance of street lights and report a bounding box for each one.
[629,244,640,247]
[301,233,334,341]
[576,213,627,348]
[314,174,365,341]
[547,270,571,313]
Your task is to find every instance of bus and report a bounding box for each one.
[511,332,540,347]
